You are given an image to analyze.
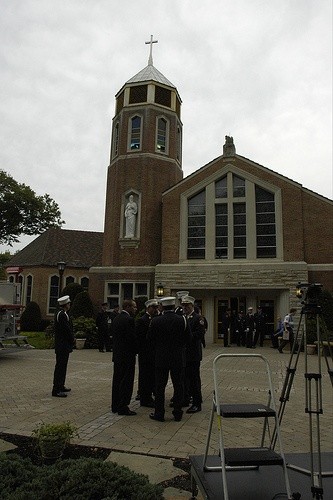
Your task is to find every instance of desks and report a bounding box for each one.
[189,451,333,500]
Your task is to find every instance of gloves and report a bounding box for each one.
[228,328,230,332]
[247,328,249,331]
[245,329,247,332]
[236,330,238,332]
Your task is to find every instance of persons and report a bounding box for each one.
[110,304,120,322]
[112,291,205,422]
[220,306,266,349]
[52,295,76,397]
[196,310,208,348]
[277,308,297,354]
[96,303,113,352]
[124,195,137,237]
[270,315,285,348]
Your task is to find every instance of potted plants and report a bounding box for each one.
[72,316,97,350]
[32,421,80,463]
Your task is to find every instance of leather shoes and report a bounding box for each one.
[61,387,71,392]
[52,392,68,397]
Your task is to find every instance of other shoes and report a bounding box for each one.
[111,390,201,421]
[99,349,104,353]
[106,349,112,352]
[278,347,283,354]
[291,350,297,354]
[249,346,255,348]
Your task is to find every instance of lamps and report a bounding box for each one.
[156,282,165,298]
[295,284,304,298]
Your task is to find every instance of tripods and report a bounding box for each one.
[269,312,333,500]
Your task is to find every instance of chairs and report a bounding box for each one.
[202,352,295,500]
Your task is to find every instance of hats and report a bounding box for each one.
[144,299,158,308]
[176,292,189,300]
[226,308,231,311]
[248,307,253,310]
[256,306,262,309]
[57,295,72,306]
[161,296,176,306]
[180,295,195,306]
[239,311,243,313]
